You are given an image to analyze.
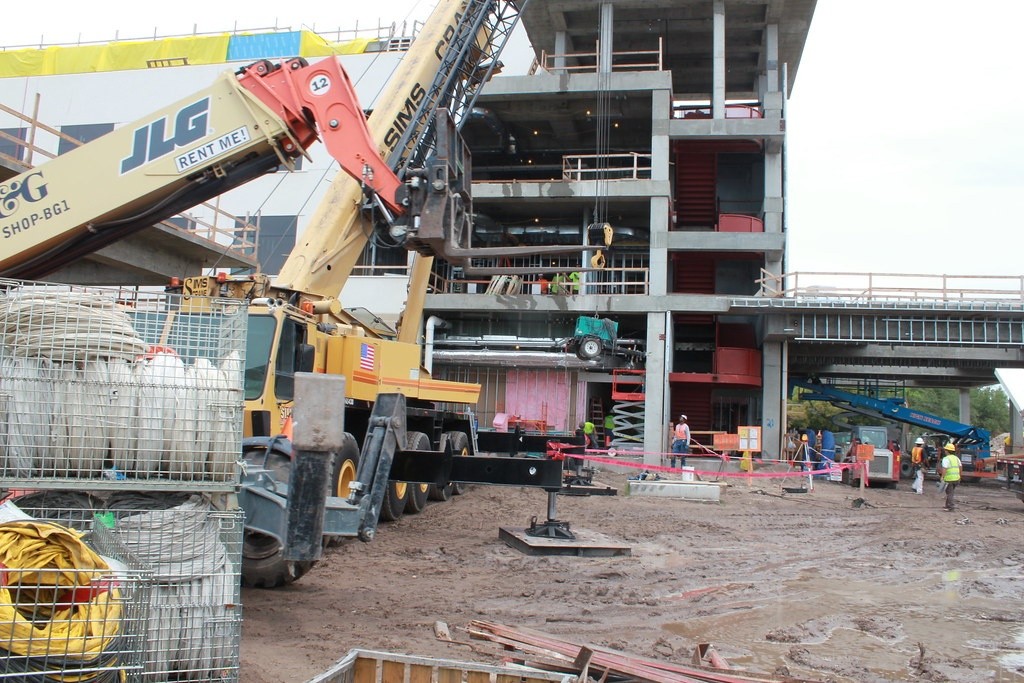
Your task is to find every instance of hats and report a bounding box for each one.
[609,409,615,413]
[681,415,687,421]
[943,444,956,451]
[915,437,925,444]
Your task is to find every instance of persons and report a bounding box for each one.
[936,442,964,512]
[578,420,604,455]
[533,272,552,294]
[567,264,582,294]
[600,409,617,446]
[666,412,691,471]
[550,268,569,293]
[909,437,930,493]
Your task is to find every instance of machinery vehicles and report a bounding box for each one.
[1,0,634,593]
[787,374,999,484]
